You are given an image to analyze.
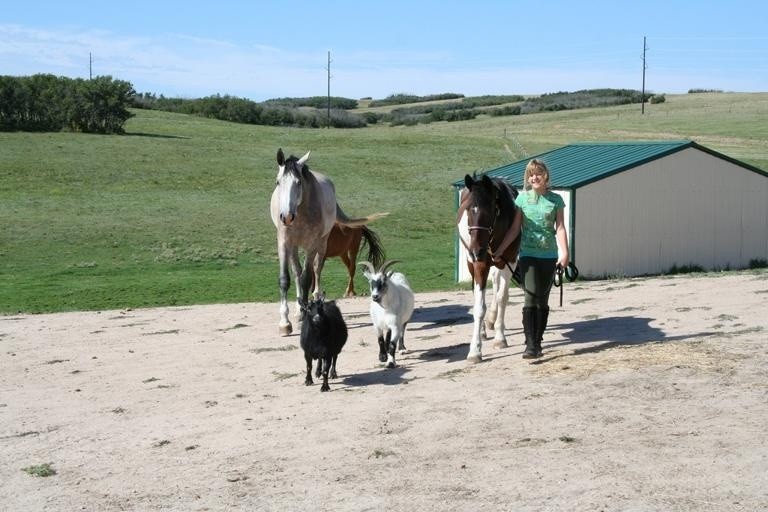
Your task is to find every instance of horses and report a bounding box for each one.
[269,147,391,337]
[456,171,522,366]
[311,222,387,299]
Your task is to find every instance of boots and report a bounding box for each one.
[523,307,537,359]
[536,307,549,356]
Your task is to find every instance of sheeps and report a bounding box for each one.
[297,291,348,393]
[357,258,415,369]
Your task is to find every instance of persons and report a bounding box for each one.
[492,159,570,358]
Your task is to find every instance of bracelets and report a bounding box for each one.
[501,242,506,249]
